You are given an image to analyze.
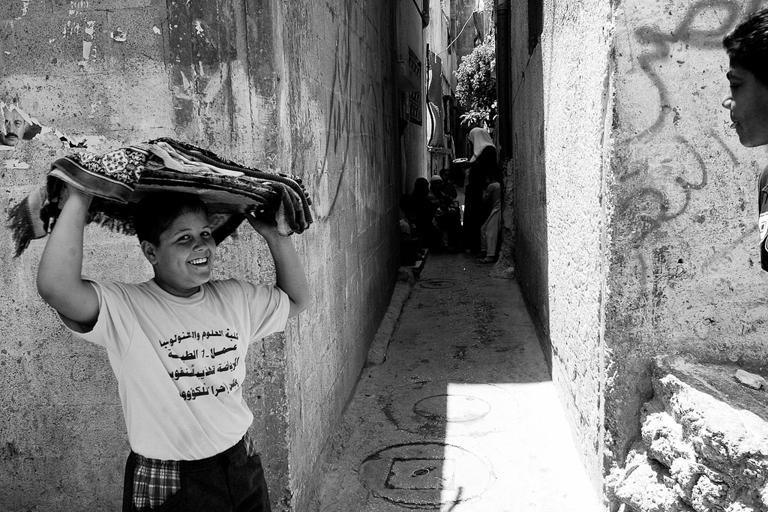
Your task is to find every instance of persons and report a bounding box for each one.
[719,7,767,276]
[34,182,310,511]
[395,127,503,265]
